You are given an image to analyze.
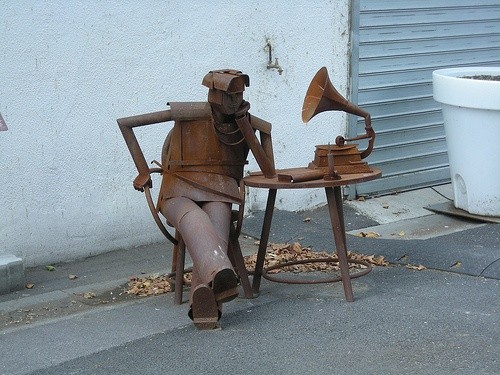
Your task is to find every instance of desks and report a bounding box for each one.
[241,167,382,303]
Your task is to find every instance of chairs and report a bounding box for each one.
[143,168,252,304]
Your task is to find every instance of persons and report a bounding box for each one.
[116,69,277,330]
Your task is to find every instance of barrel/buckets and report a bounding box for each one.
[428,64,500,218]
